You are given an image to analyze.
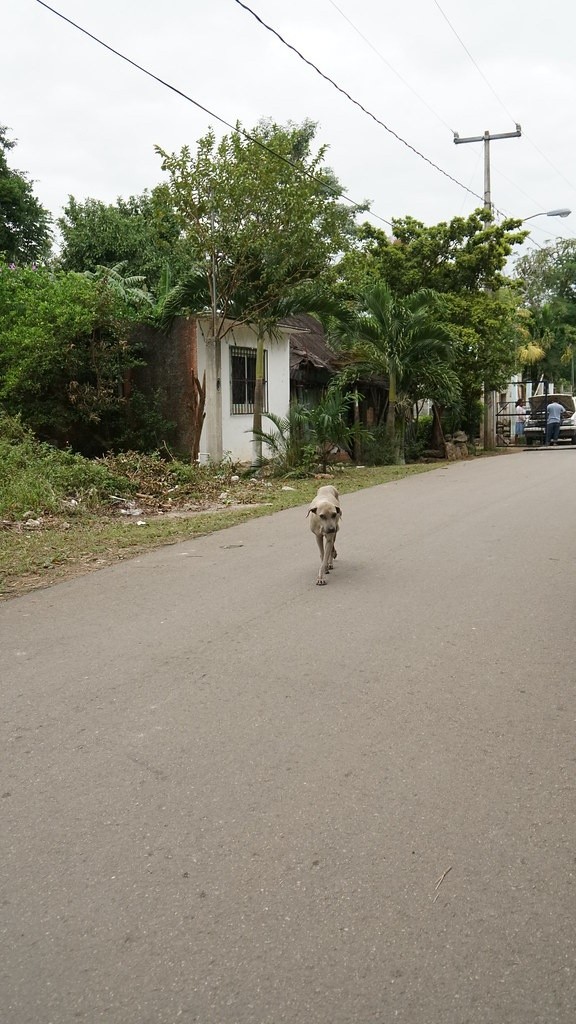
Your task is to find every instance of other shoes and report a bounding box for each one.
[552,443,558,445]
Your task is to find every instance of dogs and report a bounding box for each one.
[303,485,342,585]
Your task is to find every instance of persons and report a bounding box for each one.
[513,399,526,445]
[547,397,573,445]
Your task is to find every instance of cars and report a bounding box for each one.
[523,394,576,445]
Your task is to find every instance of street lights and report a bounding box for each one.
[481,208,572,451]
